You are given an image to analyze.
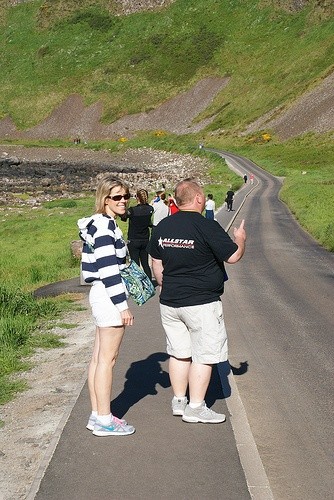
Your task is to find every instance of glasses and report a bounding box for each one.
[107,193,131,201]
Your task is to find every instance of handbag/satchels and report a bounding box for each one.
[225,198,228,202]
[119,259,155,307]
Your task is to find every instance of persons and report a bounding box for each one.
[149,190,170,280]
[244,173,247,183]
[205,193,215,220]
[250,175,254,184]
[149,178,245,423]
[167,190,178,215]
[224,188,234,211]
[120,189,154,283]
[77,176,135,436]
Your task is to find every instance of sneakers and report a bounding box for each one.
[182,401,226,423]
[92,414,136,437]
[86,412,128,431]
[171,395,188,416]
